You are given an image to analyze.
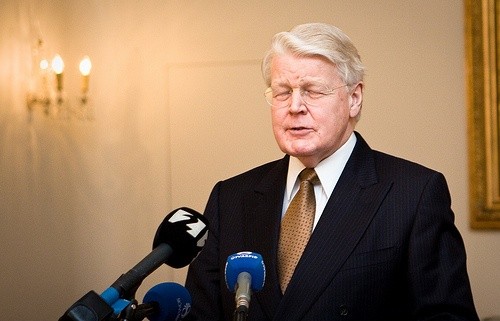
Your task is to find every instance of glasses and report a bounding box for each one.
[264,84,349,109]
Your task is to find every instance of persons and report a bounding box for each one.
[183,23,479,321]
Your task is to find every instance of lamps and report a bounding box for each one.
[29,54,91,115]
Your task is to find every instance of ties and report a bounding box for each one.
[276,168,321,296]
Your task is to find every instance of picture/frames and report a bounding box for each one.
[461,0,500,233]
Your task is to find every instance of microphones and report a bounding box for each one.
[120,281,192,321]
[224,251,267,321]
[97,206,209,305]
